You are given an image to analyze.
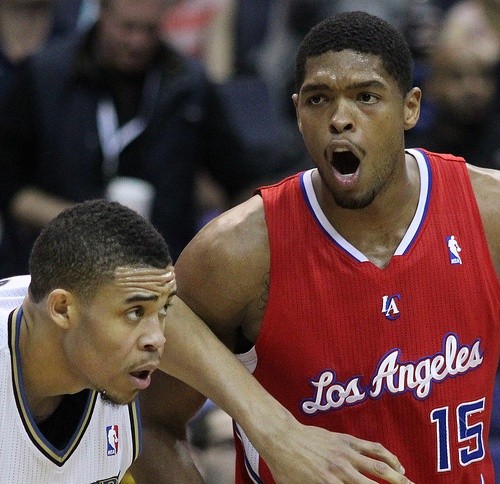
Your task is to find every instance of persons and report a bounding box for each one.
[1,0,500,269]
[1,199,177,484]
[128,10,500,484]
